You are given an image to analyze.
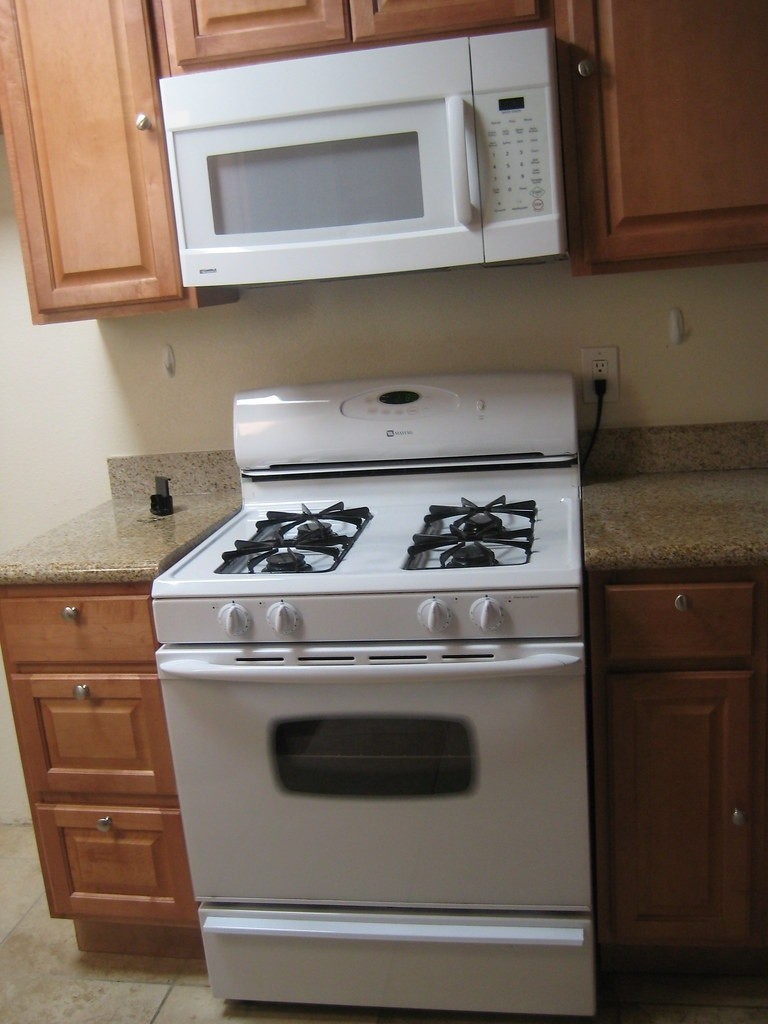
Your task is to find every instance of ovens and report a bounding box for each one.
[155,638,598,1016]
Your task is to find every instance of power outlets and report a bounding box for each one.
[581,346,620,403]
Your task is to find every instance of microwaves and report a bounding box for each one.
[160,28,570,288]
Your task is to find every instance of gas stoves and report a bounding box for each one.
[151,372,584,645]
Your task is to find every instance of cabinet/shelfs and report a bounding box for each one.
[556,0,768,276]
[584,566,768,978]
[0,1,236,327]
[156,0,556,79]
[0,581,205,959]
